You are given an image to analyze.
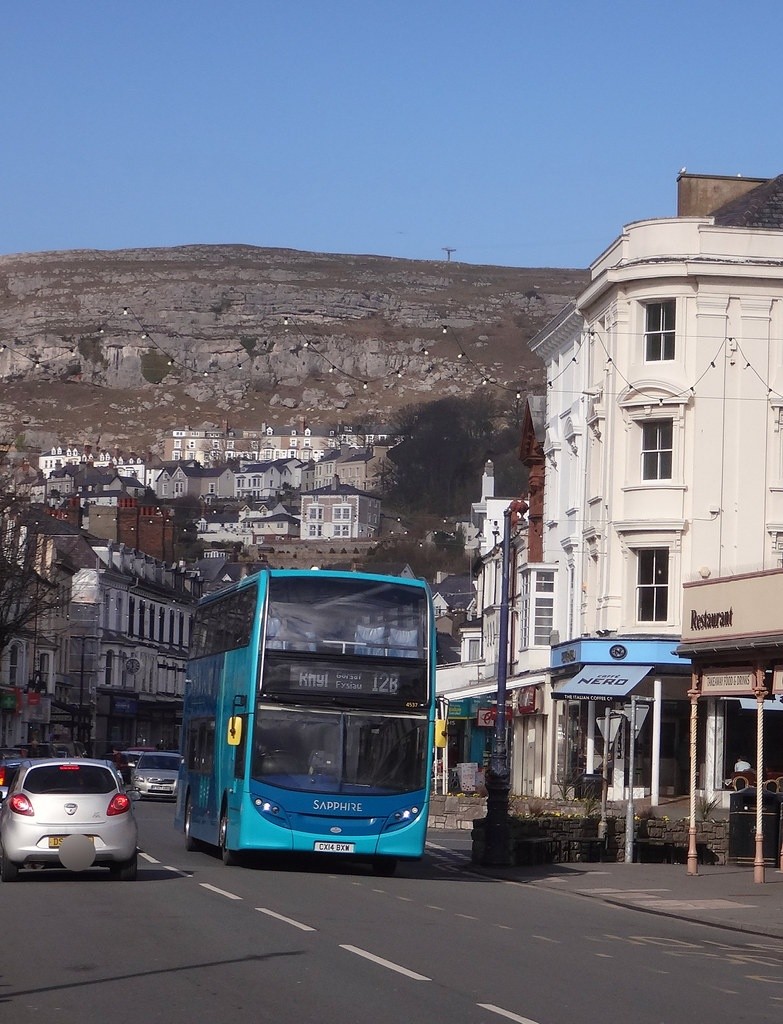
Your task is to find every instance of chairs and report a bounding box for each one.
[263,603,421,656]
[85,773,106,793]
[257,729,341,776]
[733,776,783,793]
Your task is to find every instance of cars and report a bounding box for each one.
[0,758,37,809]
[0,741,90,758]
[108,746,183,804]
[0,757,139,882]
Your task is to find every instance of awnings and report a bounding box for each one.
[551,666,655,700]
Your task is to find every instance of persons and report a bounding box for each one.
[734,754,752,772]
[111,747,130,780]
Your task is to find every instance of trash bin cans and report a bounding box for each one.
[727,786,778,868]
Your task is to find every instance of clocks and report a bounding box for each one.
[125,658,143,674]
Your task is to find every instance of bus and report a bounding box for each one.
[174,563,453,876]
